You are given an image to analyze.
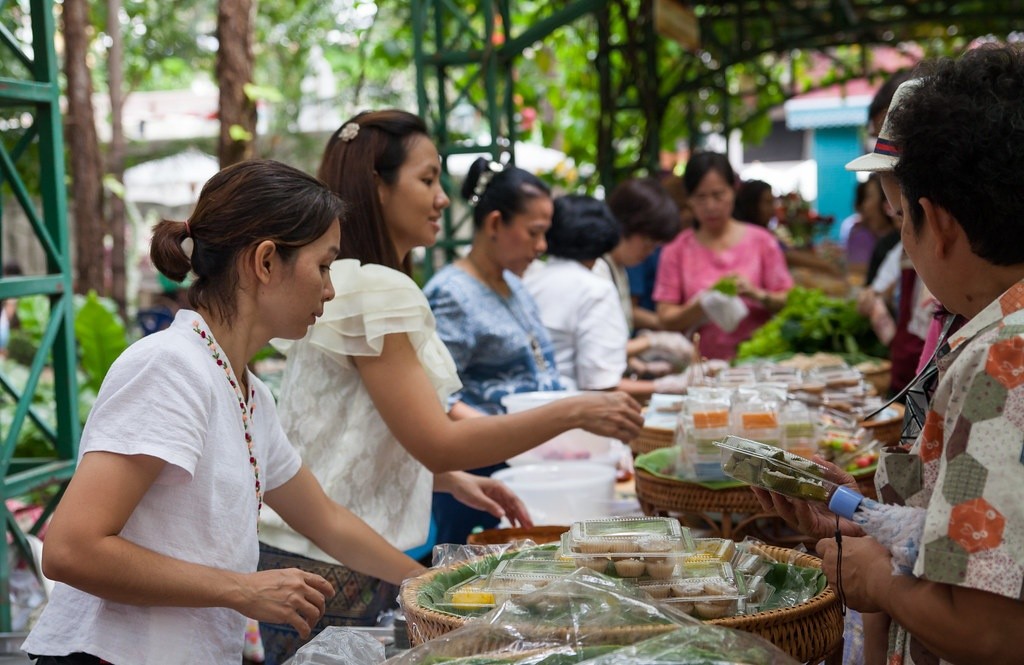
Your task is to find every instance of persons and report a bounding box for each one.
[257,110,646,665]
[752,44,1024,665]
[422,158,566,546]
[18,162,428,664]
[520,150,843,406]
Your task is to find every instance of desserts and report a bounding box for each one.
[571,536,735,622]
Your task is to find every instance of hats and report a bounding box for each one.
[846,79,932,172]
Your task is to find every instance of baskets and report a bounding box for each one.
[398,526,840,665]
[630,358,906,517]
